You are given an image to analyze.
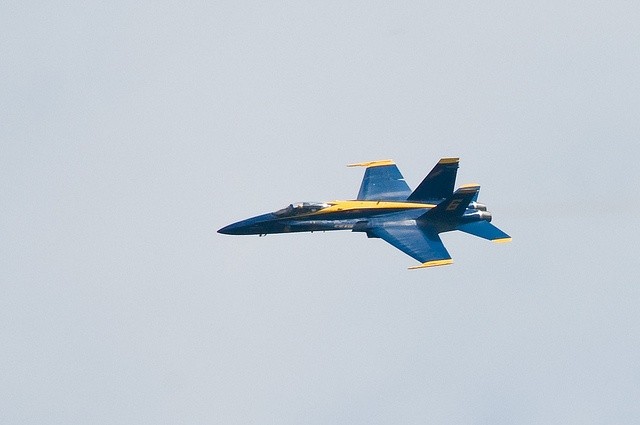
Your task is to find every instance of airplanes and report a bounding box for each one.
[216,157,514,270]
